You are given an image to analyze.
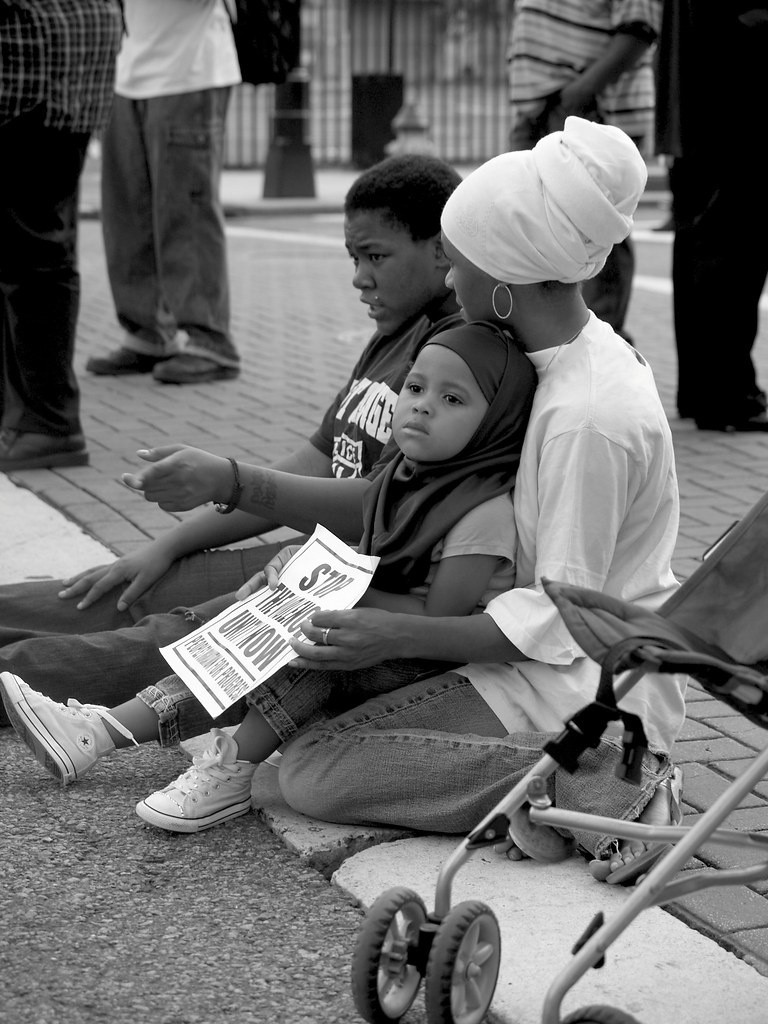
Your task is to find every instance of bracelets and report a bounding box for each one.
[213,457,242,515]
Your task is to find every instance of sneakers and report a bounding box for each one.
[136,727,259,833]
[1,671,139,788]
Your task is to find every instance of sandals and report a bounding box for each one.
[507,810,573,861]
[606,767,684,884]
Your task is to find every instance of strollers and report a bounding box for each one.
[350,491,767,1024]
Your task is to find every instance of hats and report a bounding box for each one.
[440,113,648,286]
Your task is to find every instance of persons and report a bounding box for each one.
[652,0,768,434]
[0,320,537,831]
[85,0,241,383]
[493,0,661,347]
[0,0,125,473]
[235,115,685,883]
[0,152,464,728]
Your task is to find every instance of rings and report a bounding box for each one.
[321,628,331,645]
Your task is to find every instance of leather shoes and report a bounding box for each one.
[0,430,88,470]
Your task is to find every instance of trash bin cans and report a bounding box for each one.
[352,74,404,169]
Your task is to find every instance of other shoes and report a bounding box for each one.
[153,353,238,383]
[86,348,177,377]
[676,388,768,433]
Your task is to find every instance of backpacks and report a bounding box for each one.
[234,2,301,83]
